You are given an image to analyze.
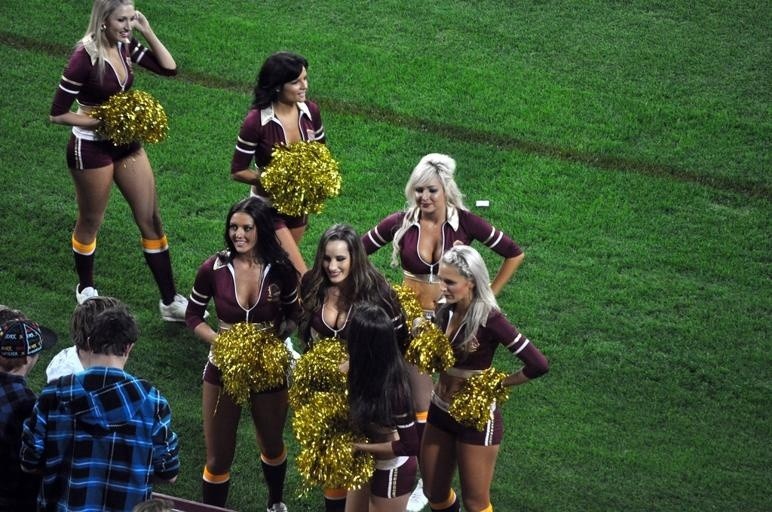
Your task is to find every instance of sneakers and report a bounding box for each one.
[159,294,210,322]
[406,478,429,512]
[76,283,99,305]
[266,502,288,512]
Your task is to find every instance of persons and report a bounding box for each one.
[0,317,58,512]
[360,153,525,512]
[18,309,181,512]
[230,52,326,367]
[49,0,210,324]
[300,224,408,512]
[344,305,420,512]
[46,295,129,384]
[184,197,302,512]
[418,245,549,512]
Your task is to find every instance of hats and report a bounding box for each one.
[0,305,58,359]
